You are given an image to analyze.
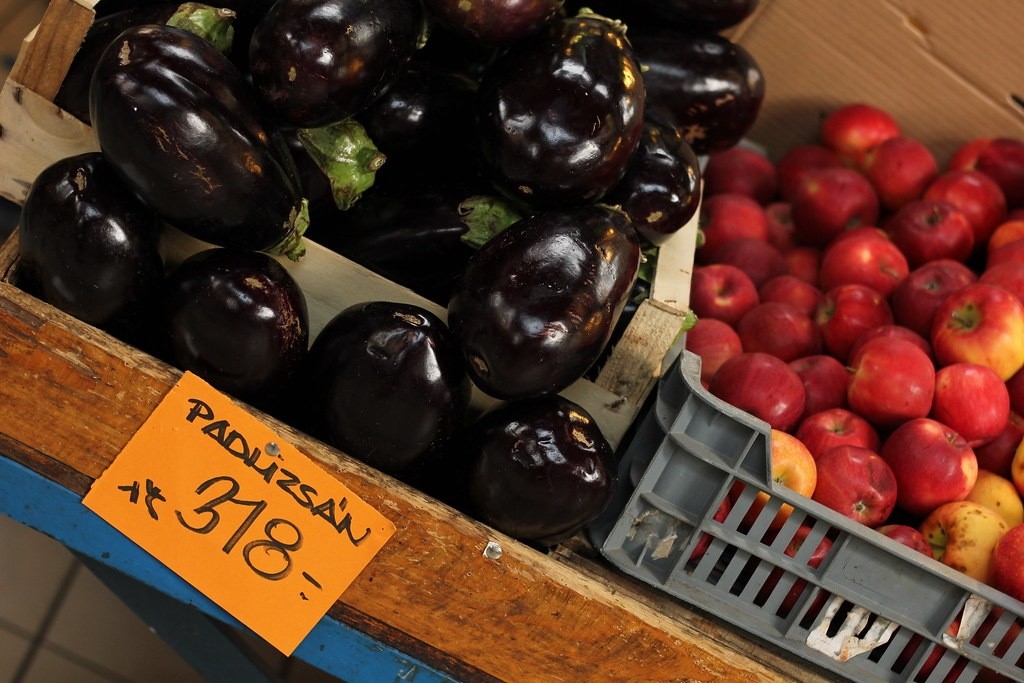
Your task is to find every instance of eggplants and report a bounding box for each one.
[13,0,766,550]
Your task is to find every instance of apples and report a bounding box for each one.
[684,105,1023,683]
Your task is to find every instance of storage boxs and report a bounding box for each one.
[0,0,1024,683]
